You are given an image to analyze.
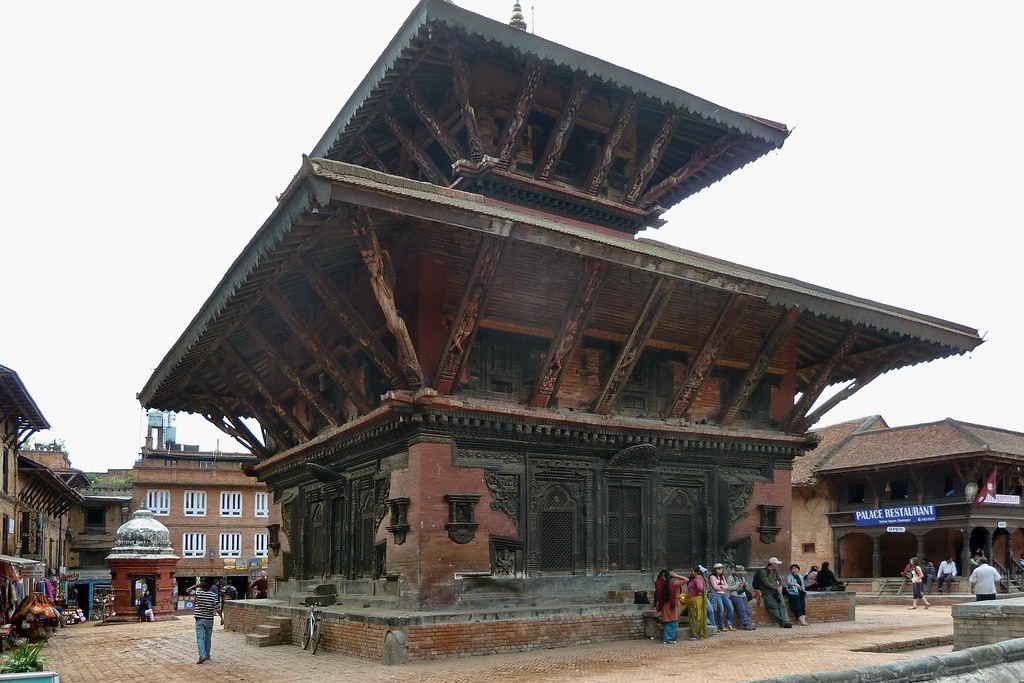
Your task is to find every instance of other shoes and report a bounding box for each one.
[924,603,930,609]
[800,621,810,626]
[725,625,737,631]
[689,636,697,641]
[782,622,792,628]
[663,639,677,644]
[718,626,728,631]
[744,624,757,630]
[907,605,916,609]
[196,656,210,664]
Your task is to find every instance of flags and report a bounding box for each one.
[974,469,996,508]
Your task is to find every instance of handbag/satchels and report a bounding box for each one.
[9,594,61,642]
[743,586,753,601]
[634,590,650,604]
[782,586,789,596]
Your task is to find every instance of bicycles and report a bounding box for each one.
[299,601,327,654]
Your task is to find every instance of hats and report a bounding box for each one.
[261,571,267,577]
[768,557,783,564]
[698,564,707,574]
[714,563,725,569]
[732,565,748,574]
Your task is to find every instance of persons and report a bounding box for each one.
[655,565,720,644]
[249,571,268,599]
[726,565,756,630]
[804,562,838,593]
[708,563,737,631]
[210,579,239,615]
[759,557,793,628]
[901,547,1024,609]
[138,589,156,622]
[187,578,224,664]
[785,564,810,626]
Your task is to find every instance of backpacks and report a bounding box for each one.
[915,565,928,584]
[752,567,780,590]
